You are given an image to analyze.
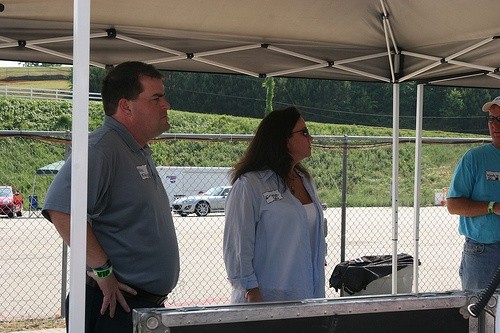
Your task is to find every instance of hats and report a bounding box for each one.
[482,95,500,112]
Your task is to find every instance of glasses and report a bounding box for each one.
[291,127,309,137]
[487,115,500,122]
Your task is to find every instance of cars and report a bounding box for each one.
[171,186,232,217]
[0,185,23,218]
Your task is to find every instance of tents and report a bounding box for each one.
[0,0,500,294]
[30,159,66,210]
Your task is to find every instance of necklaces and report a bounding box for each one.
[286,172,296,195]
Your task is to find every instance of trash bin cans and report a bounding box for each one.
[327,252,421,299]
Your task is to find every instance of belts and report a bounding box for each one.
[86,274,168,308]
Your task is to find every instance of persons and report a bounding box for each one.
[223,106,324,303]
[41,61,181,333]
[446,95,500,291]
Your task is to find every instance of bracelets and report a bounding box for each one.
[93,258,114,279]
[243,290,253,298]
[488,200,495,214]
